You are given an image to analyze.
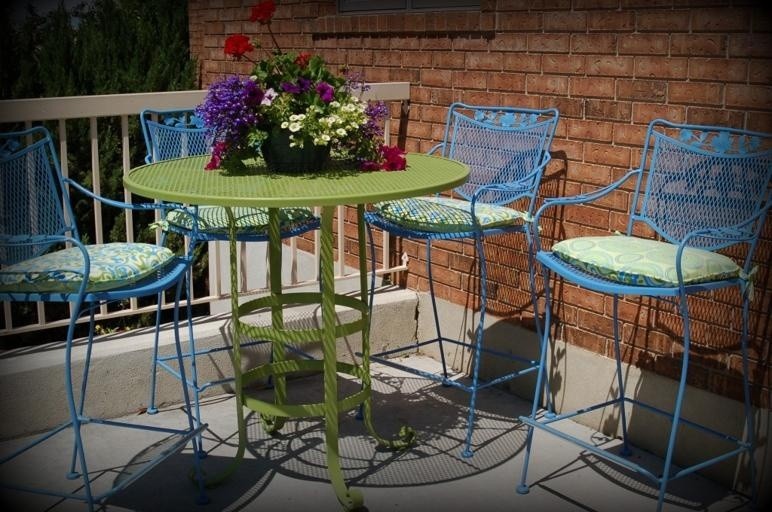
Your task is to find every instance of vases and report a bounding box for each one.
[260,126,331,176]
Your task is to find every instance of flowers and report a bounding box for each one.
[196,0,407,175]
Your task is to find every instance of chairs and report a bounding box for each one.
[0,125,206,512]
[515,119,772,512]
[355,101,559,456]
[139,107,321,458]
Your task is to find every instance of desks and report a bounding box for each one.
[122,153,471,510]
